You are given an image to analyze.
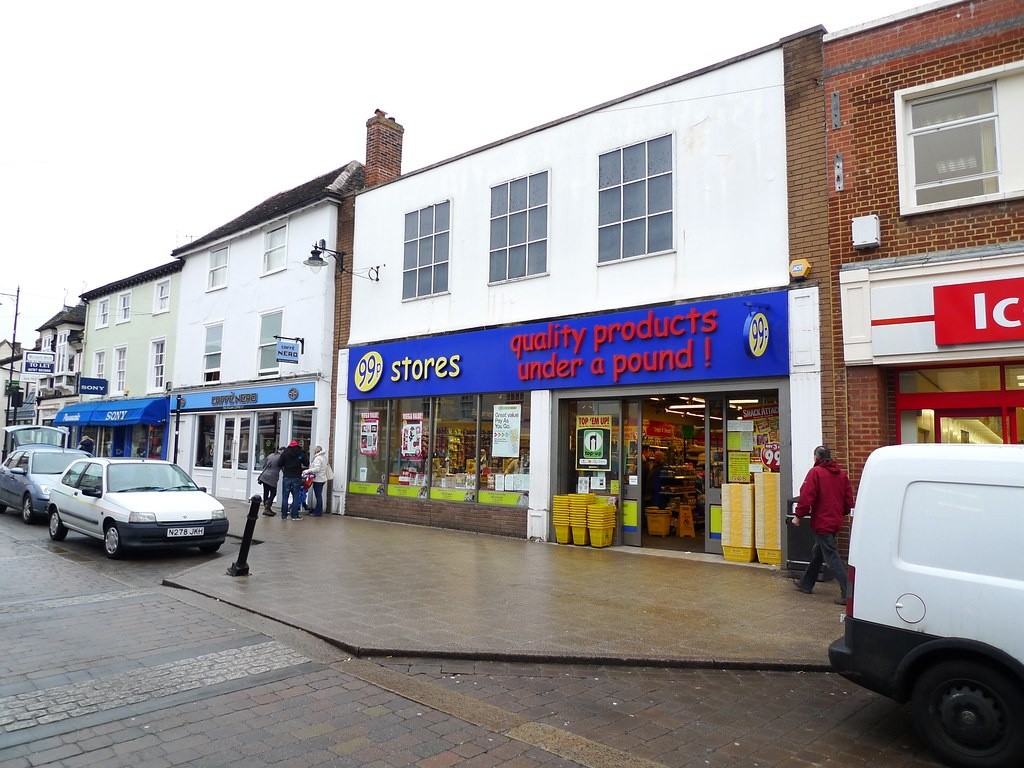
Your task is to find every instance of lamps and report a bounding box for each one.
[304,242,345,274]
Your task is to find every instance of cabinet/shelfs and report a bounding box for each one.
[421,419,724,507]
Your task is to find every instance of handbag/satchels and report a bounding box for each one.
[257,472,263,485]
[302,472,313,489]
[325,462,335,481]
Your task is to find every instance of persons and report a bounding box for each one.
[304,446,333,516]
[277,440,310,521]
[76,435,96,455]
[789,446,855,606]
[257,446,287,517]
[635,445,670,509]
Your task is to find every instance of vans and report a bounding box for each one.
[1,424,68,471]
[827,442,1024,768]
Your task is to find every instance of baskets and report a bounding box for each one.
[552,492,616,547]
[645,506,672,538]
[721,471,781,566]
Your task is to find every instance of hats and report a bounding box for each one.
[80,435,94,443]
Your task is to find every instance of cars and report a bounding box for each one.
[0,444,102,525]
[49,456,230,561]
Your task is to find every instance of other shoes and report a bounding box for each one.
[834,597,846,604]
[792,579,812,594]
[291,517,303,521]
[282,516,288,520]
[262,510,274,516]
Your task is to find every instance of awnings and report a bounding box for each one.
[52,397,168,428]
